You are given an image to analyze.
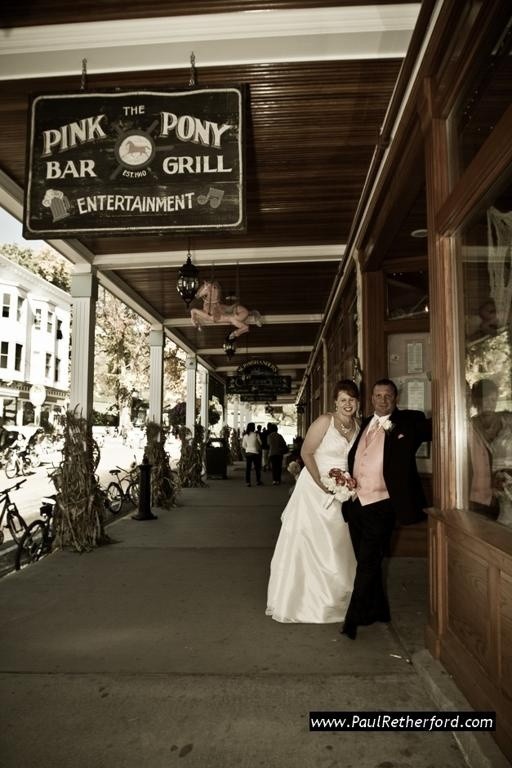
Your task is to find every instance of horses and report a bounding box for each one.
[188,279,267,350]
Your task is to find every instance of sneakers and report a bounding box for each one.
[246,481,281,488]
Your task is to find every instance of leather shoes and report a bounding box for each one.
[359,615,390,626]
[339,622,358,640]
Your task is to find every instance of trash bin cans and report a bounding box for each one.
[205,437,228,479]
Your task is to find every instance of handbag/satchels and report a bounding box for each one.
[279,442,289,454]
[255,433,262,451]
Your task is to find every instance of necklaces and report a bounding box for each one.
[339,422,353,434]
[478,420,496,435]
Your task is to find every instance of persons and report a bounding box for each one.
[470,379,511,526]
[9,432,30,476]
[188,281,263,339]
[337,378,426,639]
[263,379,362,624]
[463,381,500,521]
[240,420,302,488]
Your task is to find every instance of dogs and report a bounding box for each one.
[286,460,301,497]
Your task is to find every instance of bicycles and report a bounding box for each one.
[0,478,30,552]
[6,448,32,478]
[14,493,60,572]
[105,453,141,514]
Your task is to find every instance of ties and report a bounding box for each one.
[366,420,379,448]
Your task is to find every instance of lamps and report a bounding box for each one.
[221,326,238,365]
[174,233,201,312]
